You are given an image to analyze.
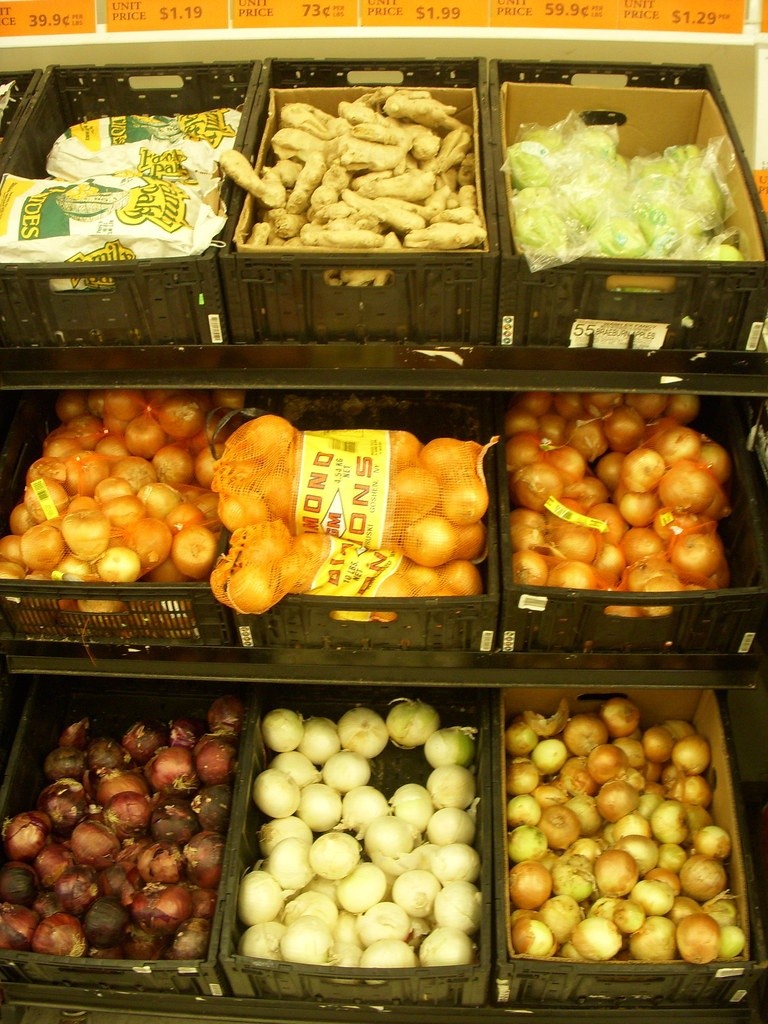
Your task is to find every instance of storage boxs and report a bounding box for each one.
[217,55,501,346]
[497,390,767,653]
[0,388,253,647]
[493,686,768,1007]
[490,57,767,352]
[0,670,256,994]
[258,388,501,652]
[0,60,262,348]
[218,682,492,1003]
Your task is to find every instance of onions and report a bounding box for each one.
[230,699,482,992]
[1,693,245,957]
[502,388,736,618]
[502,696,748,965]
[1,390,245,639]
[212,415,492,625]
